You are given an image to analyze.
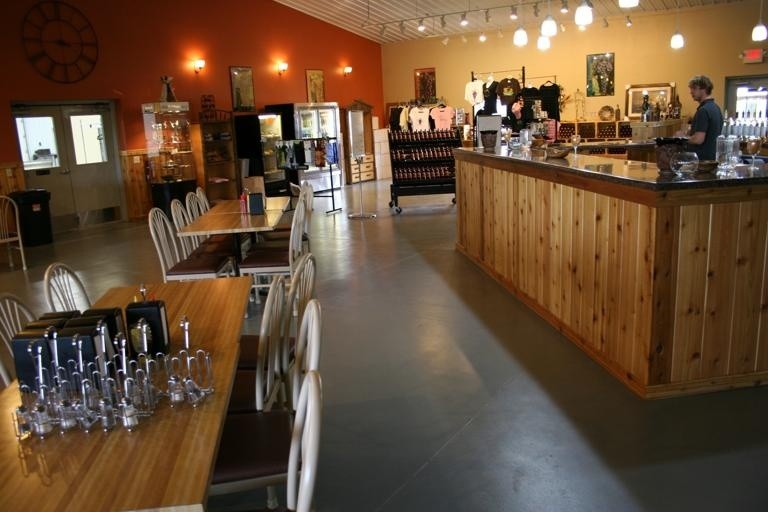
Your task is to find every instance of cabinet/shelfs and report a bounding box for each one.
[558,119,632,160]
[188,119,239,207]
[344,105,376,184]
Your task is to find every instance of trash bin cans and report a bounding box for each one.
[9,189,54,247]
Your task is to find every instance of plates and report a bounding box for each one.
[598,105,615,122]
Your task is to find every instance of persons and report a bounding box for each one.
[686,75,723,161]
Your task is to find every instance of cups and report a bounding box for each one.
[502,128,512,151]
[715,135,743,169]
[652,137,689,171]
[483,146,496,155]
[717,168,743,181]
[656,169,685,186]
[480,130,497,148]
[520,129,529,150]
[249,193,267,215]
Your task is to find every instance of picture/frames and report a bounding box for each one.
[586,52,615,97]
[414,68,436,104]
[625,82,676,122]
[229,65,256,113]
[305,68,326,102]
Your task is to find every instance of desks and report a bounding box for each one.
[177,197,290,319]
[2,277,253,511]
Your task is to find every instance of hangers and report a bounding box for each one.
[394,96,448,111]
[473,70,515,79]
[527,75,553,88]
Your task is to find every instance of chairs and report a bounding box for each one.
[148,188,248,283]
[0,263,90,388]
[240,182,314,319]
[2,196,26,271]
[203,250,324,511]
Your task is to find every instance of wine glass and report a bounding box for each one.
[570,135,582,156]
[572,155,579,168]
[746,140,761,171]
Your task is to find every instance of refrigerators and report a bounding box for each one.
[232,112,290,196]
[264,100,345,195]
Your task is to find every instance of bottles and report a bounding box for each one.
[389,127,458,179]
[639,93,682,122]
[559,124,575,139]
[578,124,595,139]
[598,125,616,138]
[721,108,767,139]
[619,125,632,137]
[616,104,620,121]
[240,188,250,214]
[583,147,626,154]
[463,113,471,124]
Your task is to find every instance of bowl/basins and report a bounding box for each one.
[509,138,523,150]
[528,149,545,158]
[545,157,571,167]
[546,146,569,158]
[532,138,554,147]
[508,151,527,159]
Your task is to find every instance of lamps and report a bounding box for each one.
[343,66,352,77]
[377,0,767,51]
[194,58,205,73]
[277,62,288,75]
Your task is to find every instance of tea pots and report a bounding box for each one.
[158,75,177,101]
